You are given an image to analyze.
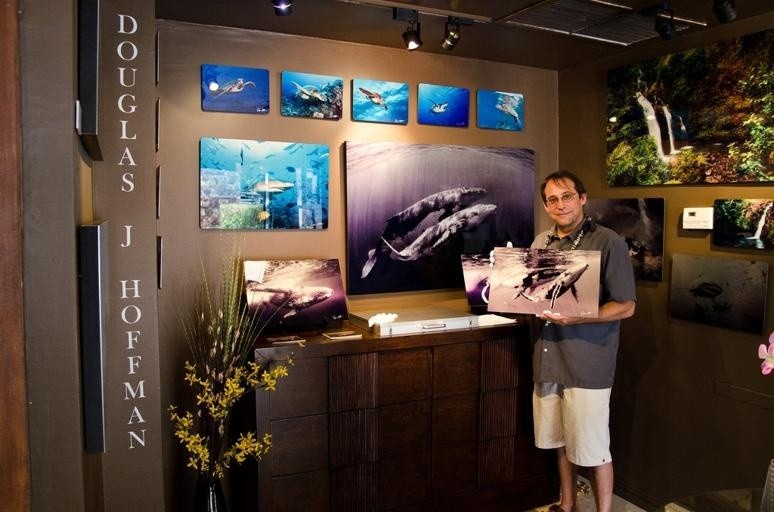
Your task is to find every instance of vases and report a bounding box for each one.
[190,477,230,512]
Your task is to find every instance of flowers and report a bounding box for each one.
[165,203,313,477]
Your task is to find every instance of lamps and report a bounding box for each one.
[403,17,462,52]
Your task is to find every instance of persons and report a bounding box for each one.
[485,169,641,511]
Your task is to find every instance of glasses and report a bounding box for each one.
[545,190,578,206]
